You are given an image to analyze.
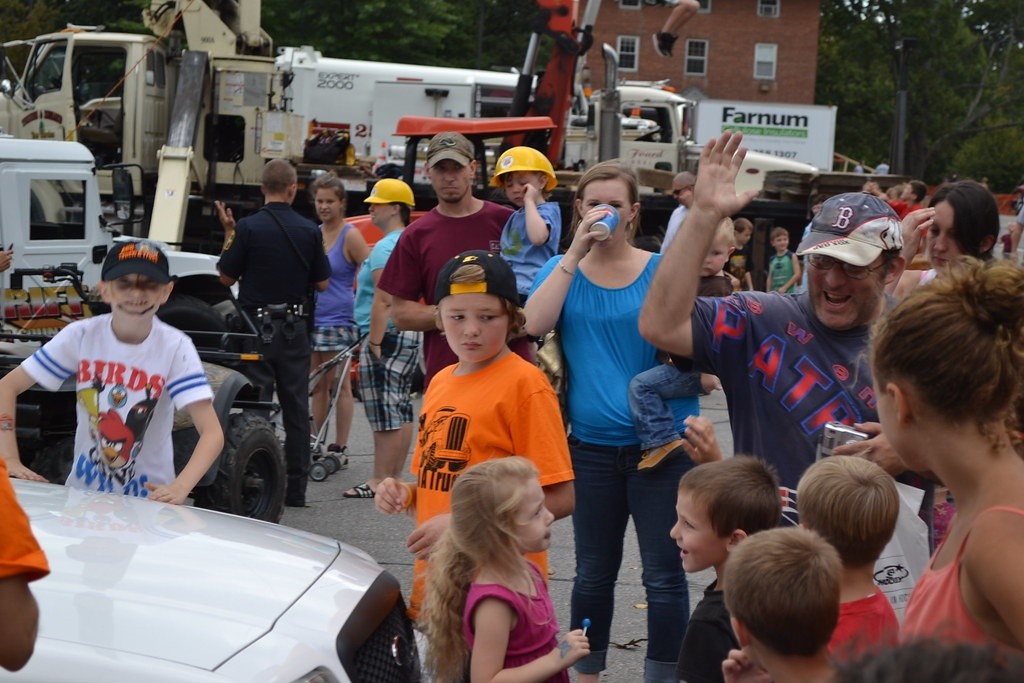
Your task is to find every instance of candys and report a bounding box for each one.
[582,618,591,636]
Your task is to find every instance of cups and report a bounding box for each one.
[588,204,621,241]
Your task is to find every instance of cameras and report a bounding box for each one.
[815,421,872,463]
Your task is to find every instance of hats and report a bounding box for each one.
[101,241,172,284]
[426,132,474,169]
[436,250,521,306]
[796,191,904,266]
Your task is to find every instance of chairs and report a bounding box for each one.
[76,108,123,148]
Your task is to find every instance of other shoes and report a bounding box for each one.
[285,498,305,507]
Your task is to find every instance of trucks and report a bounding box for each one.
[0,0,842,349]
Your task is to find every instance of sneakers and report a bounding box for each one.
[313,443,348,465]
[638,439,685,475]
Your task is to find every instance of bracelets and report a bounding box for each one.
[558,261,574,275]
[400,482,412,506]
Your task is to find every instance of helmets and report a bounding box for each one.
[364,180,415,206]
[490,147,558,191]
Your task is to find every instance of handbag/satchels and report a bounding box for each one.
[871,481,931,629]
[535,329,569,435]
[240,301,312,360]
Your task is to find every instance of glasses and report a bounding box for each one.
[809,252,889,279]
[674,184,694,194]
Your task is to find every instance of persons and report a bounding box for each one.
[341,179,418,499]
[0,128,1024,683]
[519,159,714,683]
[879,180,1001,300]
[766,227,801,294]
[723,217,754,292]
[645,0,700,57]
[629,212,735,472]
[213,158,329,508]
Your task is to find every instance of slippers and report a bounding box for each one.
[343,483,376,497]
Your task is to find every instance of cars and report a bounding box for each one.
[0,473,423,683]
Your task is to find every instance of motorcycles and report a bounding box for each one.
[0,260,290,522]
